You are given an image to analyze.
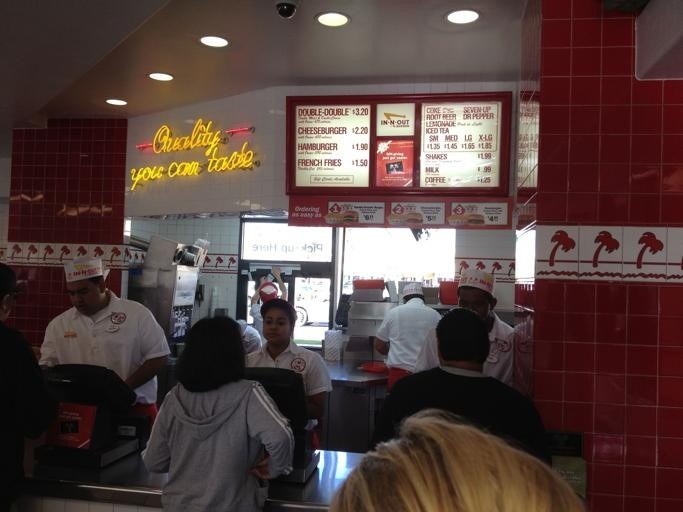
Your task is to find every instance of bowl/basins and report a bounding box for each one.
[349,289,383,302]
[352,279,383,289]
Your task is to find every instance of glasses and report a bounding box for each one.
[9,288,23,301]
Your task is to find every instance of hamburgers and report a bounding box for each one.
[468,215,485,224]
[406,213,423,224]
[343,211,359,222]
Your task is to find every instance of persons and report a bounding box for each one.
[235,320,261,354]
[38,255,172,429]
[249,266,287,336]
[244,300,332,450]
[0,261,55,511]
[369,308,553,468]
[327,409,584,512]
[375,283,443,391]
[138,316,295,512]
[412,269,514,387]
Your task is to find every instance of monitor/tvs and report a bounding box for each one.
[243,366,320,487]
[32,363,139,468]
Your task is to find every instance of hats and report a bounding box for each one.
[260,298,296,321]
[457,269,494,297]
[63,256,103,282]
[402,284,423,299]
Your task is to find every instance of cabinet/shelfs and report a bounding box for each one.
[157,357,389,453]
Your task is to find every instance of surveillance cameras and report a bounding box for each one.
[275,0,299,20]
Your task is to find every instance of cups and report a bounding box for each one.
[208,286,219,317]
